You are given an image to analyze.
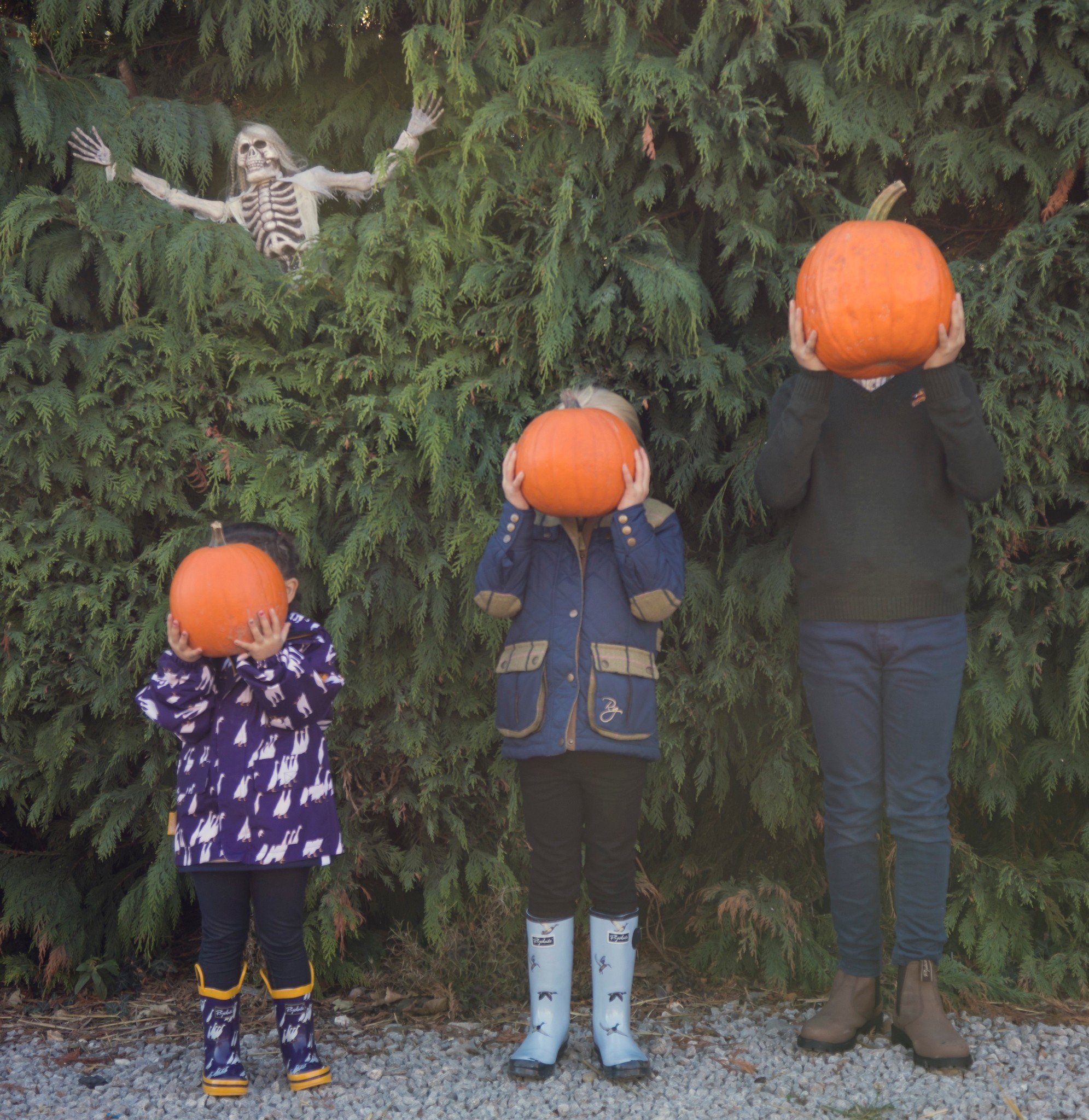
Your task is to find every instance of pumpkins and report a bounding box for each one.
[170,521,288,657]
[795,179,956,380]
[512,389,640,517]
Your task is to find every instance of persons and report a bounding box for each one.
[134,522,345,1097]
[68,88,445,282]
[755,293,1004,1067]
[472,385,687,1080]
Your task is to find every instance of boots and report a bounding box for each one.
[258,962,333,1090]
[501,910,576,1075]
[793,974,880,1048]
[585,906,651,1081]
[195,965,248,1096]
[892,959,974,1069]
